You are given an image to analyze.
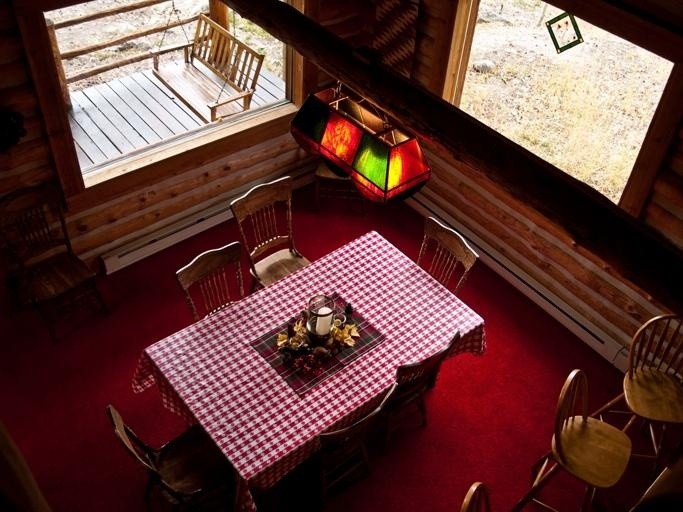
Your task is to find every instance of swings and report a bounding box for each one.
[149,1,265,124]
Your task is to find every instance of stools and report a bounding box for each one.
[310,160,371,211]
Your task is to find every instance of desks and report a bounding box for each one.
[127,224,490,510]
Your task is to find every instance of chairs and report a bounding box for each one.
[224,177,311,290]
[509,360,634,510]
[100,396,228,510]
[0,176,109,343]
[387,328,462,440]
[170,238,246,321]
[582,307,683,471]
[288,378,398,495]
[414,213,479,299]
[453,475,489,510]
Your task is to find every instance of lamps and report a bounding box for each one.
[290,70,435,209]
